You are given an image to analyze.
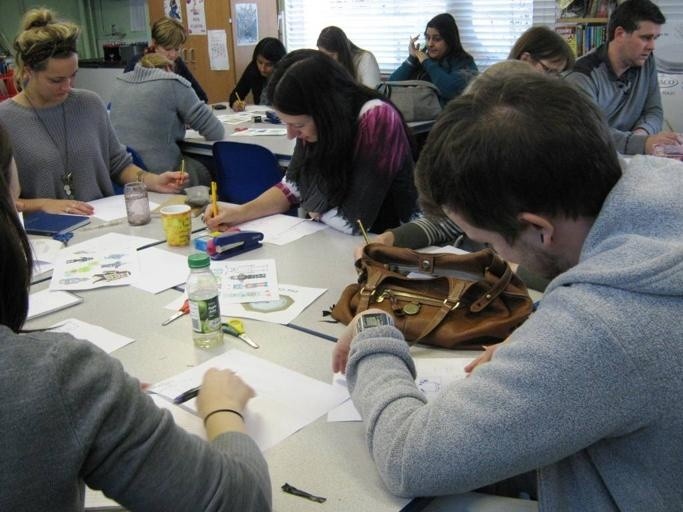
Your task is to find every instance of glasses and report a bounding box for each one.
[534,58,559,74]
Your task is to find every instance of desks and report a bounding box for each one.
[12,186,545,511]
[166,100,442,174]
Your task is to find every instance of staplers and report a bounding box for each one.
[211,230,264,261]
[265,109,281,125]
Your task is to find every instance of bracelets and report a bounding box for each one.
[135,167,149,185]
[201,408,246,429]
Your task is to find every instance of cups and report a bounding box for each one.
[122,182,150,225]
[184,185,209,205]
[159,205,190,246]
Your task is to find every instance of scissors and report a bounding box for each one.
[52,230,73,247]
[162,299,190,327]
[220,318,258,350]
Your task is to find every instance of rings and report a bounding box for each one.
[64,205,71,214]
[71,201,80,209]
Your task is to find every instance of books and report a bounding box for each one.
[554,0,623,57]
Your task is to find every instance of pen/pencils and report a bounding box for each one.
[234,89,246,112]
[174,370,237,406]
[181,158,185,185]
[211,181,217,218]
[357,219,370,245]
[665,119,674,132]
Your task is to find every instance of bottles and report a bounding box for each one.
[187,254,222,348]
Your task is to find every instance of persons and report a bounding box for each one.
[111,17,225,175]
[0,4,192,220]
[230,37,285,112]
[199,43,420,236]
[318,26,380,90]
[355,56,553,293]
[325,59,683,511]
[0,119,274,511]
[388,13,477,105]
[508,26,575,77]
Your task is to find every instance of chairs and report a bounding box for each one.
[108,145,151,195]
[207,139,299,218]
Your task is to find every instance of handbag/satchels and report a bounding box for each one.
[332,243,534,350]
[375,80,444,122]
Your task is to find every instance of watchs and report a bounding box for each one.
[352,313,395,337]
